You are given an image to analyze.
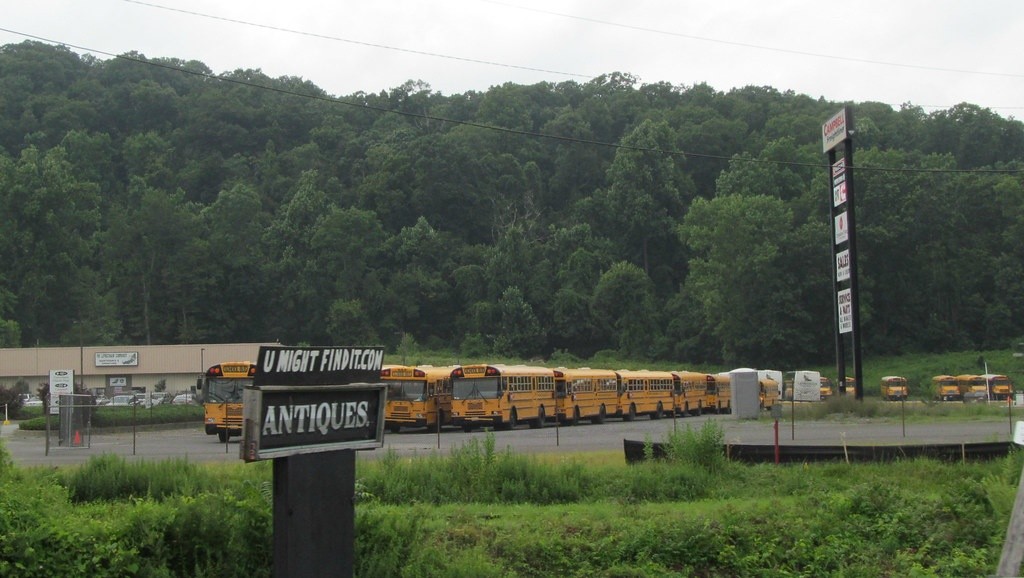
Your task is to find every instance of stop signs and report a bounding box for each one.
[510,394,515,400]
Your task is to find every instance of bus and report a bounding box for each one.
[615,370,674,422]
[704,372,732,413]
[931,375,960,401]
[672,371,708,417]
[957,375,988,400]
[982,374,1013,400]
[881,375,908,402]
[202,362,257,443]
[380,365,463,433]
[554,367,617,425]
[846,376,855,396]
[785,377,834,400]
[759,379,778,410]
[450,364,556,433]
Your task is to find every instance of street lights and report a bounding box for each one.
[73,321,83,395]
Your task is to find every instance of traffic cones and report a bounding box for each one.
[74,431,81,446]
[1007,396,1011,403]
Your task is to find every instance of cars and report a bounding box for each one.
[19,392,201,410]
